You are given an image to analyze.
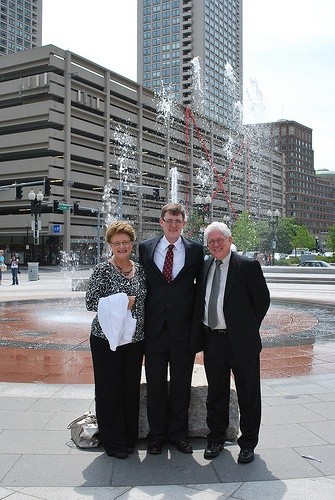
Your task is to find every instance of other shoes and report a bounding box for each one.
[127,447,133,453]
[113,450,128,458]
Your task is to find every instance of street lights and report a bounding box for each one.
[193,193,213,245]
[267,208,282,265]
[27,188,44,262]
[315,236,320,253]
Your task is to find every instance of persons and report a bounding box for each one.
[137,201,203,455]
[192,221,271,464]
[85,220,146,459]
[0,249,21,286]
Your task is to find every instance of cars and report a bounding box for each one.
[294,261,335,269]
[237,247,335,266]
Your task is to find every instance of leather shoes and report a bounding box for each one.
[148,440,162,454]
[204,444,223,457]
[175,440,192,452]
[238,449,254,463]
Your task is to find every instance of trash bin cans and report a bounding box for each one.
[27,262,39,281]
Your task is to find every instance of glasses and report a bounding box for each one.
[110,240,131,246]
[162,217,183,225]
[207,237,228,245]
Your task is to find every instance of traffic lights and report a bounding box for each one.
[16,186,23,199]
[74,203,79,215]
[53,199,60,213]
[44,179,52,195]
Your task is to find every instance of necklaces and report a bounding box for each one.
[112,257,134,277]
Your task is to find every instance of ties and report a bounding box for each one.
[162,244,175,283]
[208,260,223,331]
